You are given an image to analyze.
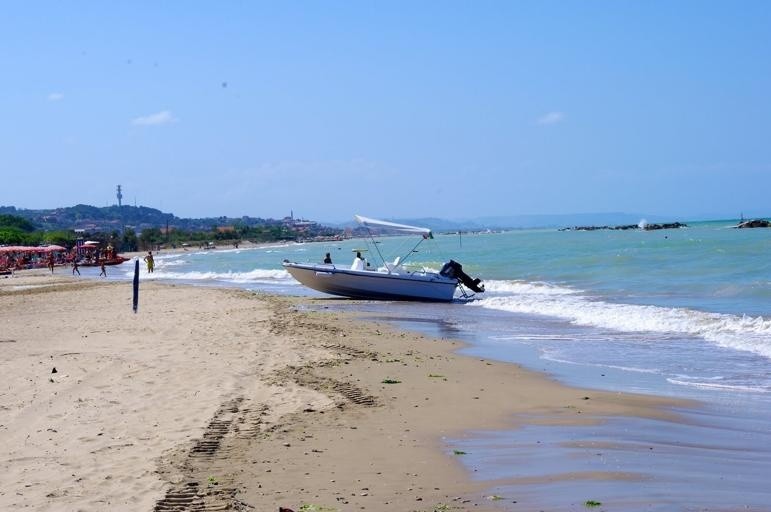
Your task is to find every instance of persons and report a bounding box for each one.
[144,251,155,273]
[72,261,80,275]
[46,253,55,274]
[323,253,336,269]
[351,252,363,270]
[99,261,107,277]
[0,245,117,268]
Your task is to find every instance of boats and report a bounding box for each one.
[736,220,768,227]
[77,245,124,266]
[282,215,459,302]
